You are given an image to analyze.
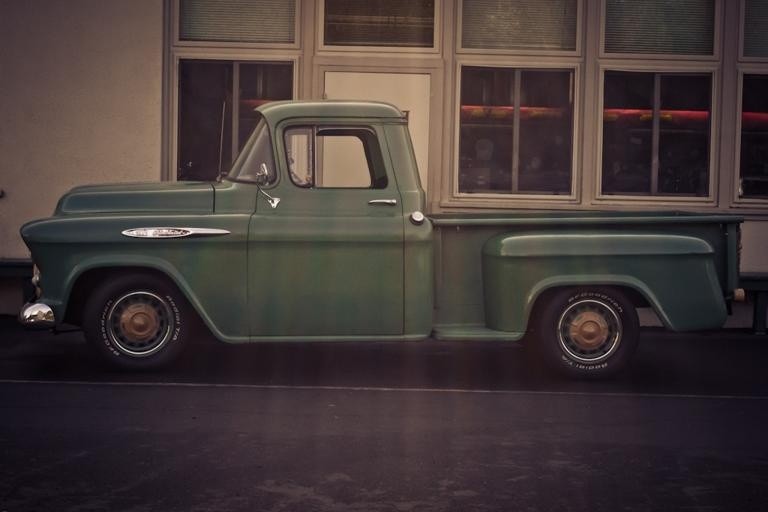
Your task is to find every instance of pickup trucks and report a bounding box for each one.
[13,97,748,387]
